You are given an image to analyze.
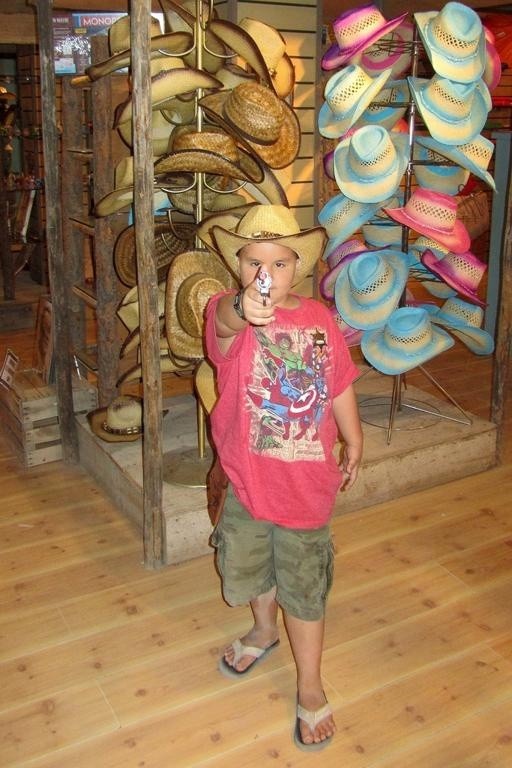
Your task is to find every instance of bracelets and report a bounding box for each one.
[233,289,246,321]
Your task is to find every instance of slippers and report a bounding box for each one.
[294,687,334,751]
[219,636,280,679]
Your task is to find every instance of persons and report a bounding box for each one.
[183,203,366,753]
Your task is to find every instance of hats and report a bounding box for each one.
[317,2,496,375]
[85,0,327,416]
[86,394,170,443]
